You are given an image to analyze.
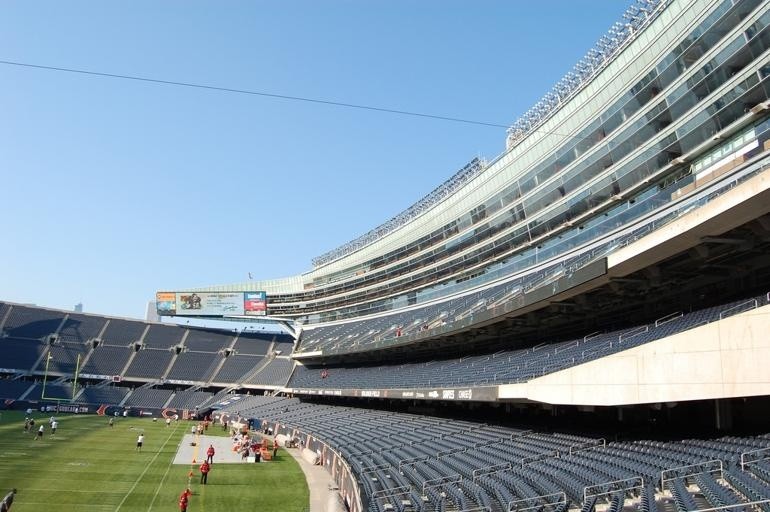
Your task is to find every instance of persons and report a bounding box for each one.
[442,320,446,326]
[396,325,401,336]
[133,433,144,453]
[423,321,429,329]
[0,487,17,512]
[49,418,60,439]
[199,459,210,485]
[25,404,78,415]
[173,413,179,424]
[180,488,191,512]
[196,422,203,436]
[166,416,171,428]
[33,423,45,441]
[191,424,197,437]
[49,415,56,424]
[22,417,30,434]
[28,418,35,431]
[272,440,278,456]
[206,444,215,464]
[123,409,128,418]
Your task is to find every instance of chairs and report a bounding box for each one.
[244,293,769,512]
